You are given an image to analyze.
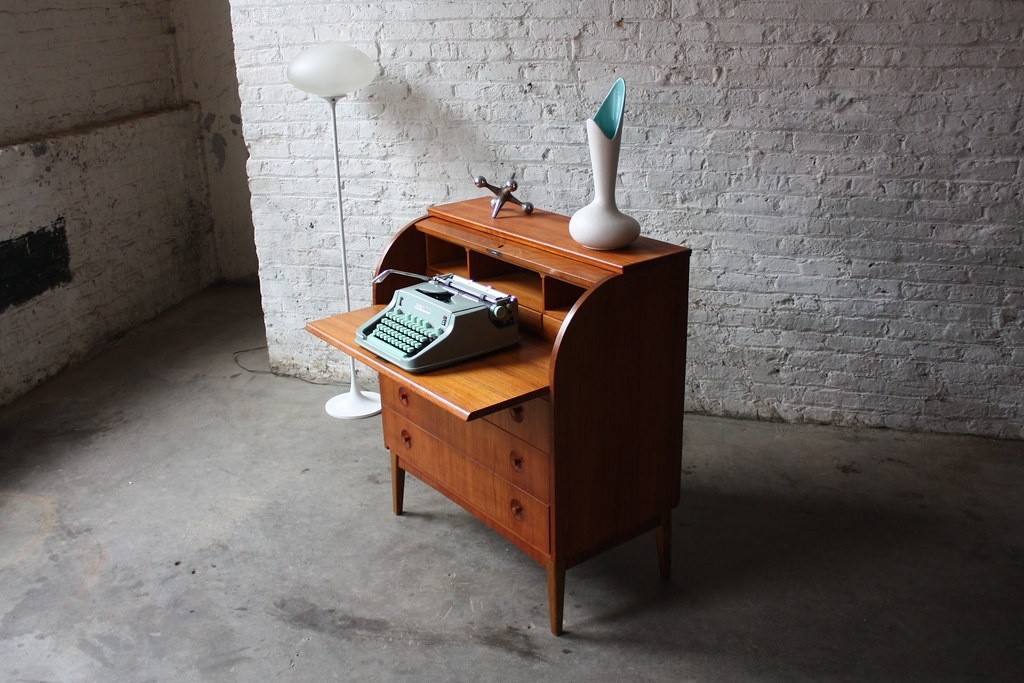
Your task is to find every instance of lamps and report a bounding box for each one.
[284,35,397,421]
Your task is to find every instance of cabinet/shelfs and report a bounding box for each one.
[299,194,694,636]
